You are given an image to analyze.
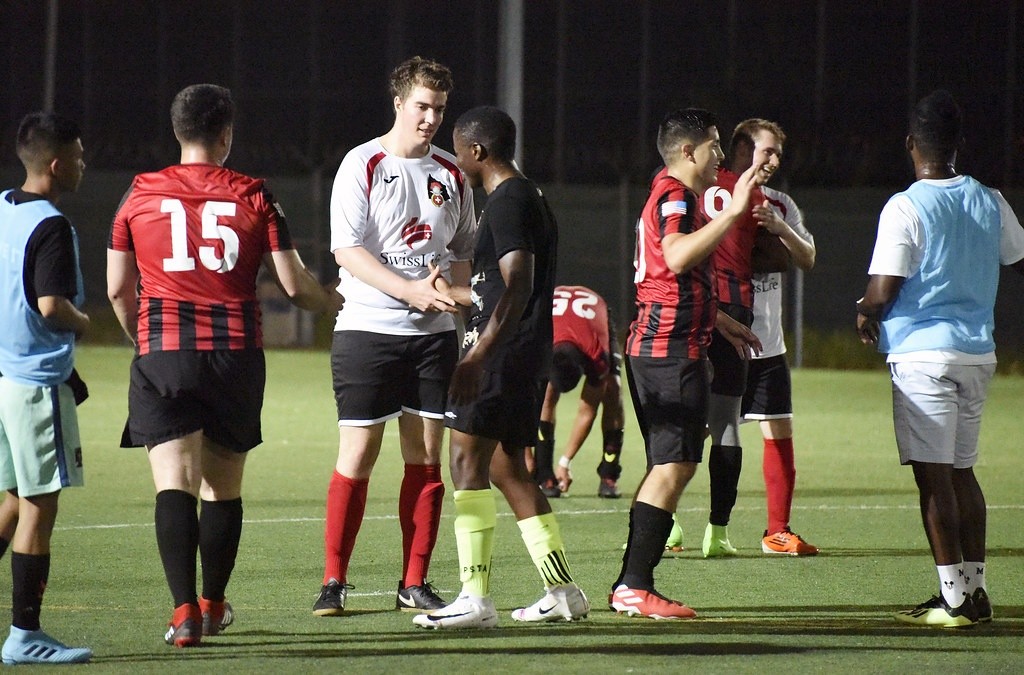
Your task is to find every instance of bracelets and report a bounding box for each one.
[855,297,881,319]
[559,456,571,469]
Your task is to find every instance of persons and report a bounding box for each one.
[526,286,624,498]
[107,84,318,646]
[408,105,590,630]
[611,111,760,621]
[856,98,1024,627]
[313,56,477,614]
[0,110,93,662]
[622,119,818,557]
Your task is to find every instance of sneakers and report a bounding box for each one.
[164,603,204,648]
[761,526,819,555]
[893,587,993,628]
[511,588,590,622]
[2,625,91,665]
[313,577,355,616]
[622,513,685,552]
[539,479,561,498]
[395,577,450,614]
[702,523,737,558]
[598,478,621,498]
[412,592,497,630]
[608,583,699,621]
[198,595,234,636]
